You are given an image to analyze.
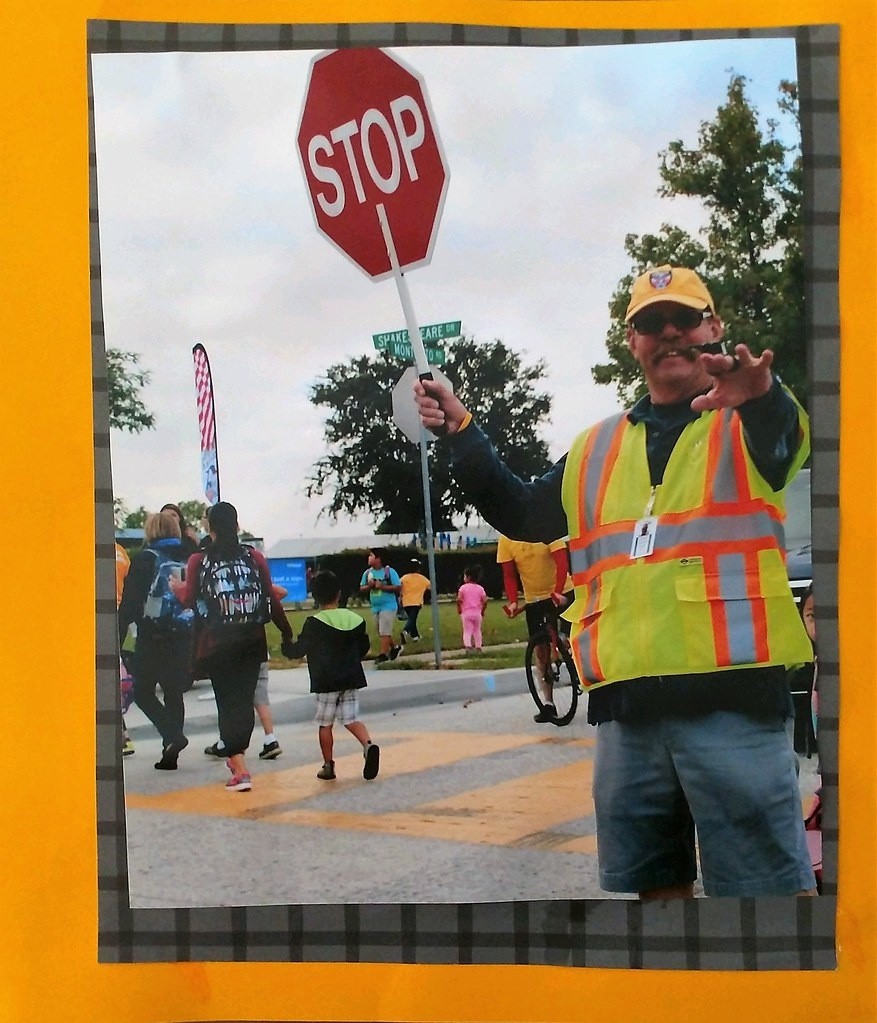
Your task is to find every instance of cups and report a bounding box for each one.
[170,567,183,581]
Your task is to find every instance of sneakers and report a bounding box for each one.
[225,772,252,791]
[389,645,403,661]
[362,741,380,780]
[226,757,236,774]
[375,654,388,665]
[318,760,336,779]
[260,741,282,759]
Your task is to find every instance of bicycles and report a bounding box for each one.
[501,594,586,727]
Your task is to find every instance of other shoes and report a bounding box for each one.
[400,631,407,643]
[164,737,188,760]
[154,762,178,770]
[413,635,419,643]
[534,705,557,722]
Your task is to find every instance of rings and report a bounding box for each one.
[729,354,740,373]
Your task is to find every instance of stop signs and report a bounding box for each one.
[295,44,453,283]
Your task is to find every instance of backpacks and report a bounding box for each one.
[195,544,271,630]
[143,548,195,633]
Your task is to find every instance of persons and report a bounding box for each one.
[638,524,649,537]
[118,513,207,771]
[458,566,488,653]
[399,560,431,643]
[280,570,380,780]
[497,532,575,725]
[115,503,283,760]
[359,548,402,665]
[796,580,821,822]
[413,266,822,899]
[169,503,283,792]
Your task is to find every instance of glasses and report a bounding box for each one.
[630,308,712,336]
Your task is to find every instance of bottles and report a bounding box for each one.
[558,631,572,658]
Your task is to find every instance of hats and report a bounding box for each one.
[624,264,715,325]
